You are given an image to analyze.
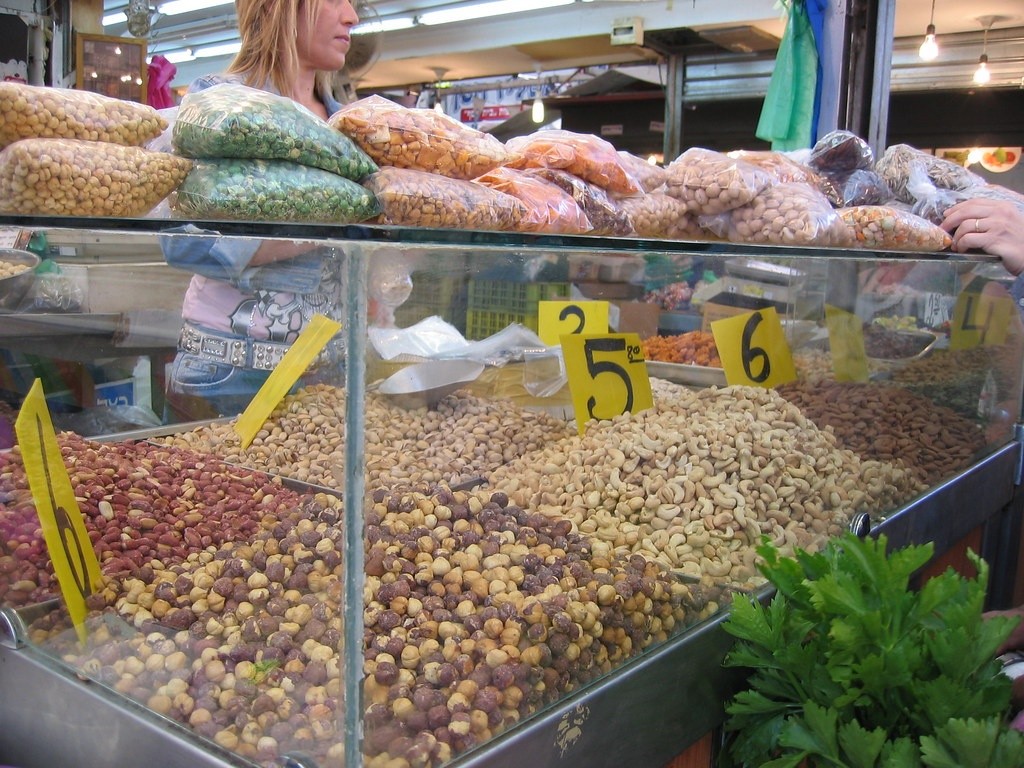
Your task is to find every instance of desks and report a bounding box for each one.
[0,333,178,423]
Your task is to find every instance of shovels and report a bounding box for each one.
[365,359,485,410]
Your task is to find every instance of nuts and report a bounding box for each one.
[668,149,953,253]
[1,333,1014,768]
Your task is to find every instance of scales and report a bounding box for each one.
[691,258,808,315]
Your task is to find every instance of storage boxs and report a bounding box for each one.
[18,375,137,415]
[399,277,568,355]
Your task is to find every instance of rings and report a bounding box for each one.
[975,218,979,232]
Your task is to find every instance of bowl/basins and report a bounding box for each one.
[0,250,41,310]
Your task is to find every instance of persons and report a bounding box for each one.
[158,0,359,427]
[938,198,1024,332]
